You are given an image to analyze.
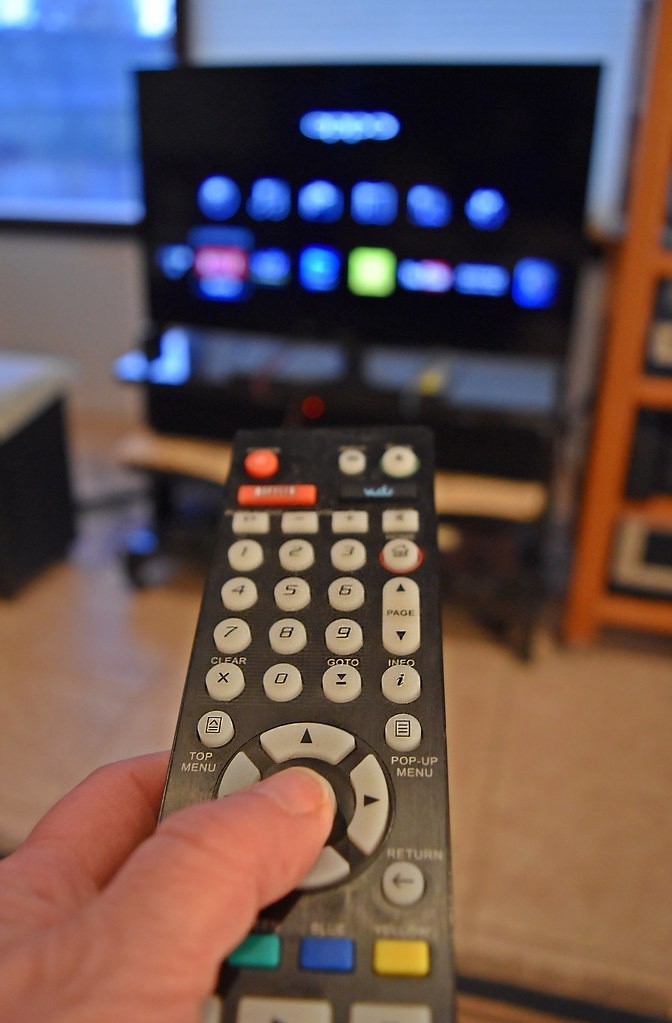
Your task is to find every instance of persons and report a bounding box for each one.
[0,749,335,1023]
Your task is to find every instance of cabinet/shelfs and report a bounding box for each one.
[564,0,671,643]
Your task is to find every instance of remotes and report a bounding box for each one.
[165,423,457,1023]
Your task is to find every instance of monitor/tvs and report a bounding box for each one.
[132,63,601,362]
